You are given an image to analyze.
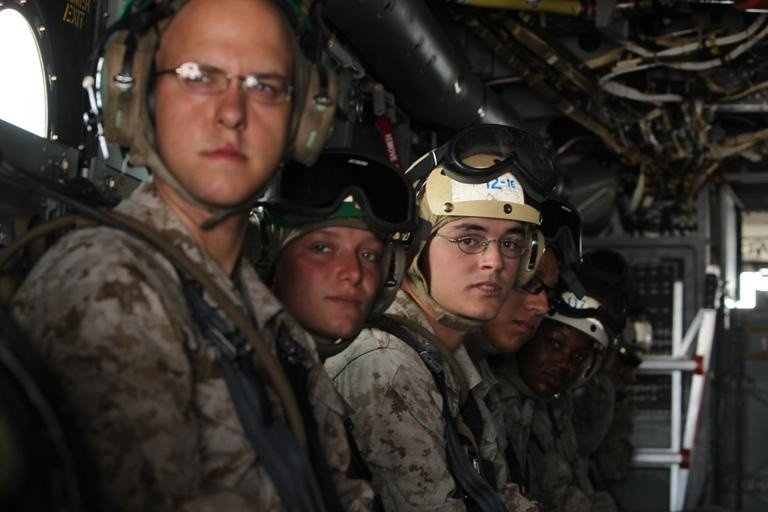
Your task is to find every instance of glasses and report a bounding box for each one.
[523,274,557,299]
[444,123,561,203]
[156,63,292,105]
[438,232,528,258]
[263,148,414,229]
[559,282,627,334]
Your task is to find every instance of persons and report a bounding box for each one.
[491,241,638,511]
[306,124,539,511]
[460,194,586,511]
[248,146,420,366]
[571,336,644,481]
[1,1,380,511]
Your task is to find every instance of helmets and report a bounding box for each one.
[247,146,404,323]
[545,291,608,379]
[404,144,546,287]
